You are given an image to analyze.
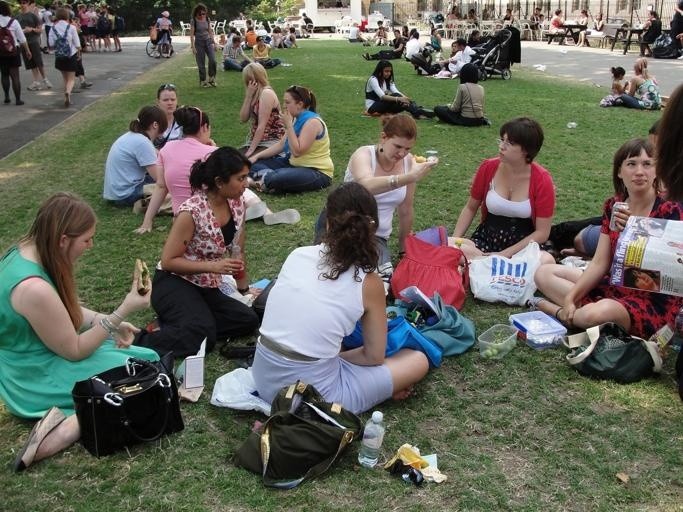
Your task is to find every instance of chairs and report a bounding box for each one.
[252,20,277,33]
[333,20,351,35]
[431,20,447,38]
[514,17,533,42]
[180,21,191,37]
[448,22,507,43]
[216,20,227,35]
[305,21,315,38]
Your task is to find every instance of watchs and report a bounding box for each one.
[238,286,249,294]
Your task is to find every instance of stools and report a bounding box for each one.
[611,38,639,53]
[545,33,591,47]
[637,41,655,56]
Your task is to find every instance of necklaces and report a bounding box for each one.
[501,164,525,192]
[375,149,395,172]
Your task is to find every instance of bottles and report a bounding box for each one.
[608,202,629,231]
[358,411,385,468]
[231,245,245,280]
[426,151,438,167]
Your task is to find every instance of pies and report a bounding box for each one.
[133,258,150,295]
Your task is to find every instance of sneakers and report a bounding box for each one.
[64,91,68,107]
[244,201,266,221]
[201,82,208,87]
[43,78,53,88]
[104,47,107,51]
[578,43,584,46]
[15,406,66,476]
[28,81,40,90]
[362,54,367,59]
[4,99,9,102]
[108,48,110,51]
[71,88,79,93]
[209,81,217,86]
[576,42,581,46]
[81,80,92,87]
[99,47,102,51]
[264,208,301,225]
[366,52,371,59]
[16,101,24,105]
[564,40,567,44]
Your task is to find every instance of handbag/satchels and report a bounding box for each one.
[389,291,476,358]
[254,278,279,322]
[566,320,663,385]
[464,82,492,128]
[132,184,173,216]
[344,317,443,373]
[389,235,469,311]
[72,352,185,459]
[232,379,363,491]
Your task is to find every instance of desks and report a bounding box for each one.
[611,28,671,55]
[558,25,586,45]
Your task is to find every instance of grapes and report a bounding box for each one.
[484,328,514,357]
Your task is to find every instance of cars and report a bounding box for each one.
[600,17,629,36]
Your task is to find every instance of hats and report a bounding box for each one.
[161,10,169,17]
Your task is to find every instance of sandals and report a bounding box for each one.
[526,297,544,309]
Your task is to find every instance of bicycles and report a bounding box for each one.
[145,26,174,59]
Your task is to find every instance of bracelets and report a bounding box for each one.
[238,287,250,295]
[387,175,399,190]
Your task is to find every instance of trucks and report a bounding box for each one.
[304,0,390,32]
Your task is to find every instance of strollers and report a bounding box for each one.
[468,27,522,80]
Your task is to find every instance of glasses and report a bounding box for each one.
[197,12,206,16]
[291,85,304,103]
[159,83,175,91]
[193,106,203,137]
[258,38,263,41]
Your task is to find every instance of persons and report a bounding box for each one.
[0,191,160,473]
[188,3,314,87]
[103,106,169,207]
[576,13,604,47]
[405,33,424,60]
[504,9,514,27]
[365,61,428,119]
[251,182,429,418]
[452,118,556,265]
[448,6,489,25]
[673,3,683,44]
[316,115,430,273]
[560,117,665,257]
[434,63,485,127]
[549,9,569,45]
[0,0,123,106]
[410,28,416,37]
[247,86,335,194]
[150,11,172,58]
[611,66,629,97]
[372,21,388,45]
[377,27,385,45]
[639,11,662,56]
[655,82,683,402]
[237,62,285,164]
[131,105,301,235]
[449,41,459,56]
[468,30,486,46]
[349,23,362,42]
[526,139,683,339]
[573,10,588,43]
[622,59,662,110]
[539,14,546,28]
[134,145,258,357]
[529,8,541,29]
[402,25,408,41]
[153,84,184,150]
[448,38,477,79]
[364,30,404,61]
[425,28,444,53]
